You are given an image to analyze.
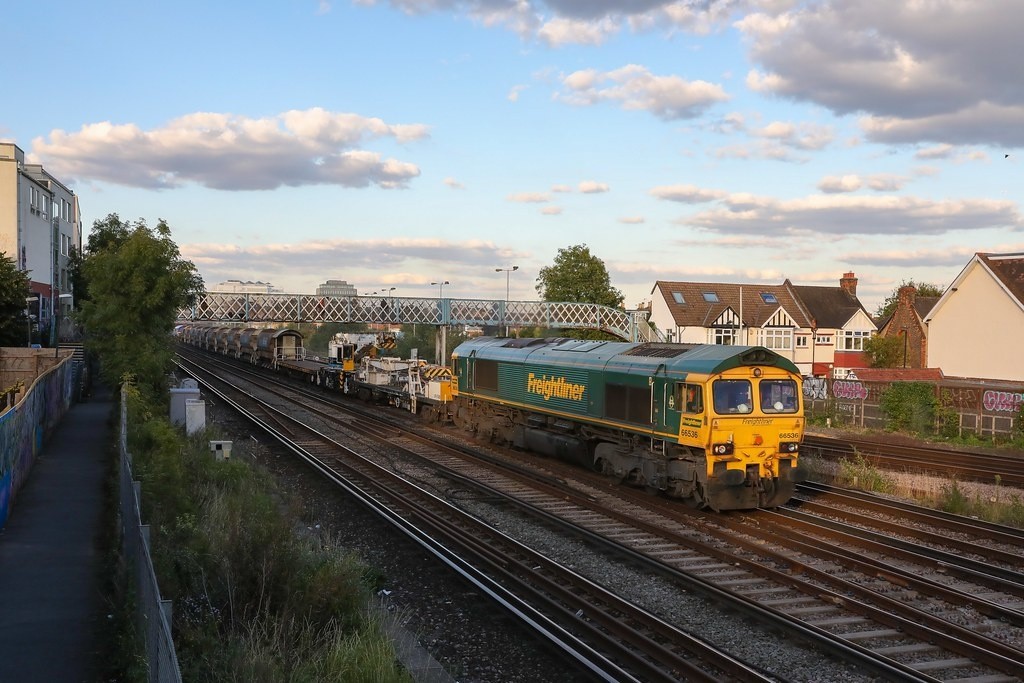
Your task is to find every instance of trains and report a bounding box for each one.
[175,320,807,511]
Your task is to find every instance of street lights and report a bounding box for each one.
[492,266,518,301]
[428,281,450,296]
[365,291,376,319]
[494,266,520,301]
[382,286,397,317]
[26,297,40,349]
